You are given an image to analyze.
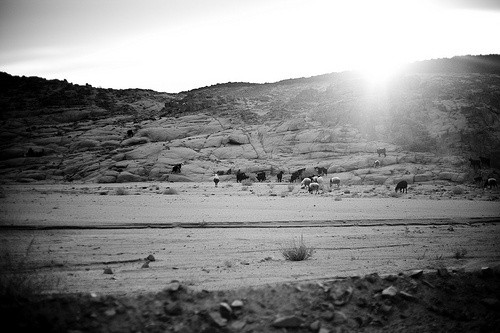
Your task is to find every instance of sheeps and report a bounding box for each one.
[277,171,285,182]
[289,167,328,195]
[330,177,340,187]
[256,172,266,182]
[395,181,408,194]
[377,148,386,157]
[171,162,249,183]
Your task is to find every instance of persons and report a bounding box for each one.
[237,169,241,183]
[213,174,219,187]
